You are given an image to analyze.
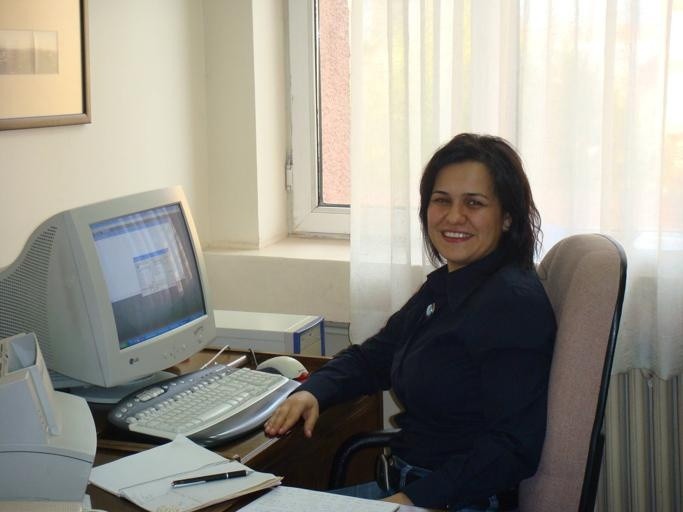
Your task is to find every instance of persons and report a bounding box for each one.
[263,133,557,512]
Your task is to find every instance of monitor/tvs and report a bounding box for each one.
[0,183,216,406]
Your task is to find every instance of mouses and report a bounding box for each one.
[257,356,312,380]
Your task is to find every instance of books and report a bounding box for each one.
[88,433,284,512]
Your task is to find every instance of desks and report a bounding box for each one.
[55,346,447,512]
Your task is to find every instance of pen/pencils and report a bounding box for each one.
[171,470,255,488]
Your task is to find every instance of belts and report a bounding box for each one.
[374,446,519,510]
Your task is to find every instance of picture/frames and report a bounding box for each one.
[0,0,91,132]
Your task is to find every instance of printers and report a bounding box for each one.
[0,331,99,503]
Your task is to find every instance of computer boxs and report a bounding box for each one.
[205,310,326,358]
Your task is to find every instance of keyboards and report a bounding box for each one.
[105,361,302,452]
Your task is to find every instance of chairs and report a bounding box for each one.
[329,231,626,511]
